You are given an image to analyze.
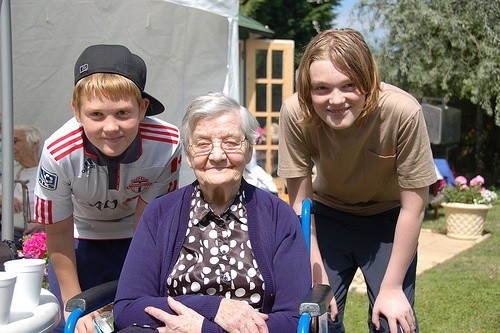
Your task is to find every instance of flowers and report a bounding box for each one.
[15,231,50,259]
[429,176,498,208]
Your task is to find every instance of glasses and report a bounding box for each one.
[187,137,247,155]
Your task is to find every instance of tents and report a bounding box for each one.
[0,0,240,243]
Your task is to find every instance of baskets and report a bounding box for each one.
[441,202,493,240]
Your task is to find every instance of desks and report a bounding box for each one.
[427,159,456,218]
[0,286,62,333]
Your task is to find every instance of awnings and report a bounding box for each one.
[238,13,275,36]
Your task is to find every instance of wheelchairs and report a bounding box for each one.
[64,197,334,333]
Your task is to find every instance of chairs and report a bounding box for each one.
[62,197,334,333]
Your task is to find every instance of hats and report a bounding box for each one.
[74,44,165,116]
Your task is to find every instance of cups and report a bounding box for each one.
[0,272,17,325]
[4,259,46,309]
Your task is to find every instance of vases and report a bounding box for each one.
[440,202,492,240]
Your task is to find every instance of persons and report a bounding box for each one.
[113,91,312,333]
[33,44,182,333]
[277,27,438,333]
[0,124,43,250]
